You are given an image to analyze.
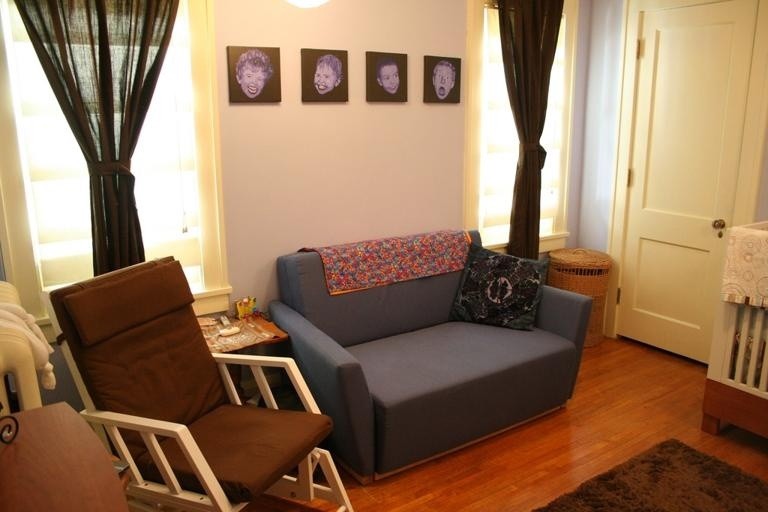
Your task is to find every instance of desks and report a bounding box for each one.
[191,297,291,358]
[0,402,130,512]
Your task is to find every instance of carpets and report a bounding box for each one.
[530,431,768,512]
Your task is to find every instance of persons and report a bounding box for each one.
[231,48,274,98]
[429,59,457,100]
[374,57,401,96]
[311,53,344,96]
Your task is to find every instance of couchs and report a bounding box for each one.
[270,229,594,487]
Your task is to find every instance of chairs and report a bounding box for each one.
[40,254,358,511]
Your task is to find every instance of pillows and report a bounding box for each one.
[447,240,551,333]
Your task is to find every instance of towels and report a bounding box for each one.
[720,220,768,310]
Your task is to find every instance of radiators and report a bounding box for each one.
[0,274,48,413]
